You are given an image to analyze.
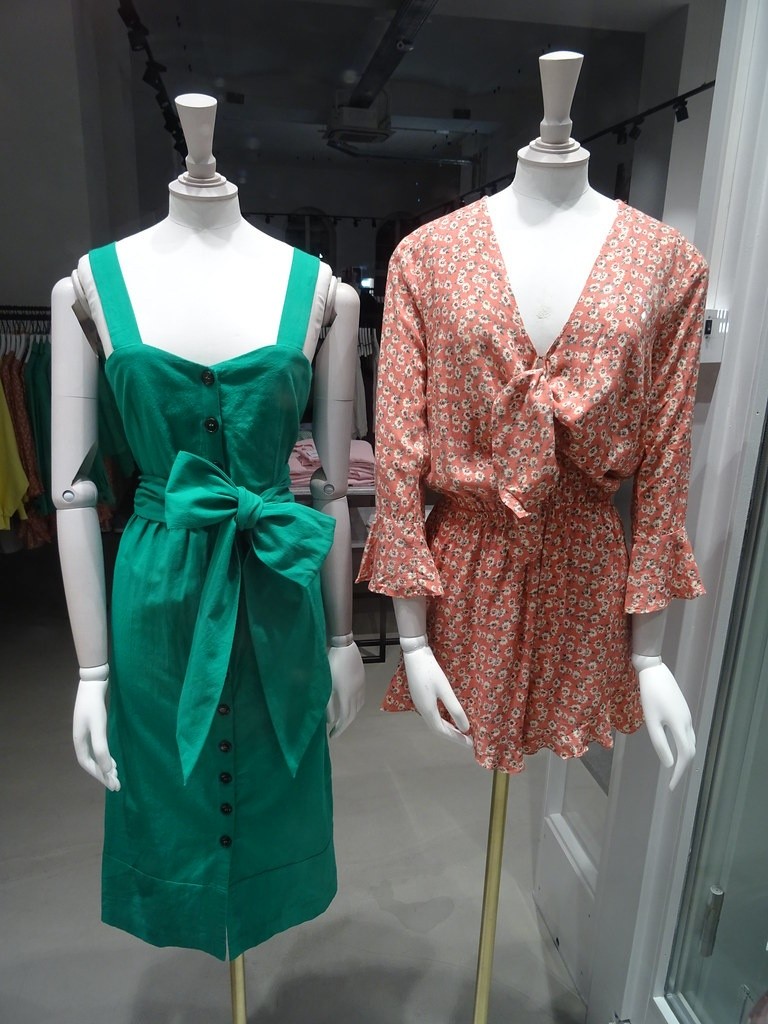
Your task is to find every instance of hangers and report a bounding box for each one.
[319,295,385,356]
[0,305,51,363]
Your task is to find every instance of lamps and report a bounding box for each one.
[128,30,145,52]
[612,126,626,144]
[672,100,689,123]
[629,119,643,139]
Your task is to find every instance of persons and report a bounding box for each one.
[376,147,704,799]
[47,166,359,965]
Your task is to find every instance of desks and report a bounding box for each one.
[290,488,430,664]
